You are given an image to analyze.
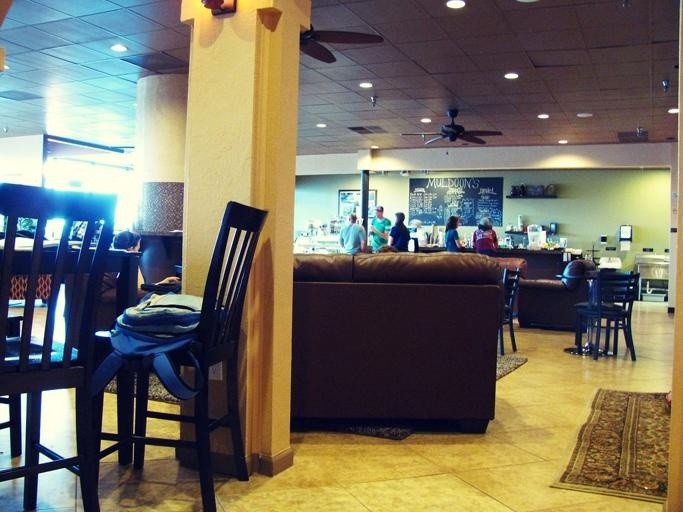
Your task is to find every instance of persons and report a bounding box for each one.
[95,231,180,330]
[340,206,498,254]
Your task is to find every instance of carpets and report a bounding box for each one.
[548,386,671,504]
[87,355,530,442]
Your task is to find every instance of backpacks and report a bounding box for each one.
[86,292,229,400]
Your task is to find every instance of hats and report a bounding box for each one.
[480,217,492,226]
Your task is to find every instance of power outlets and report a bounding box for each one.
[203,362,223,381]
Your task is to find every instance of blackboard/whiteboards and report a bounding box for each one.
[408,178,503,227]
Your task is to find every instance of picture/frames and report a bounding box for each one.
[338,189,377,219]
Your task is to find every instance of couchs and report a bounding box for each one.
[292,251,501,434]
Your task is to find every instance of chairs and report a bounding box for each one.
[91,200,268,511]
[0,185,116,511]
[500,267,522,355]
[517,259,595,334]
[574,270,640,361]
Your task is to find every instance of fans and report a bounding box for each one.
[299,24,383,66]
[399,107,503,145]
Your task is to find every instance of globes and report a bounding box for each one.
[556,260,600,292]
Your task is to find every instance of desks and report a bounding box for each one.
[555,273,626,355]
[0,248,145,489]
